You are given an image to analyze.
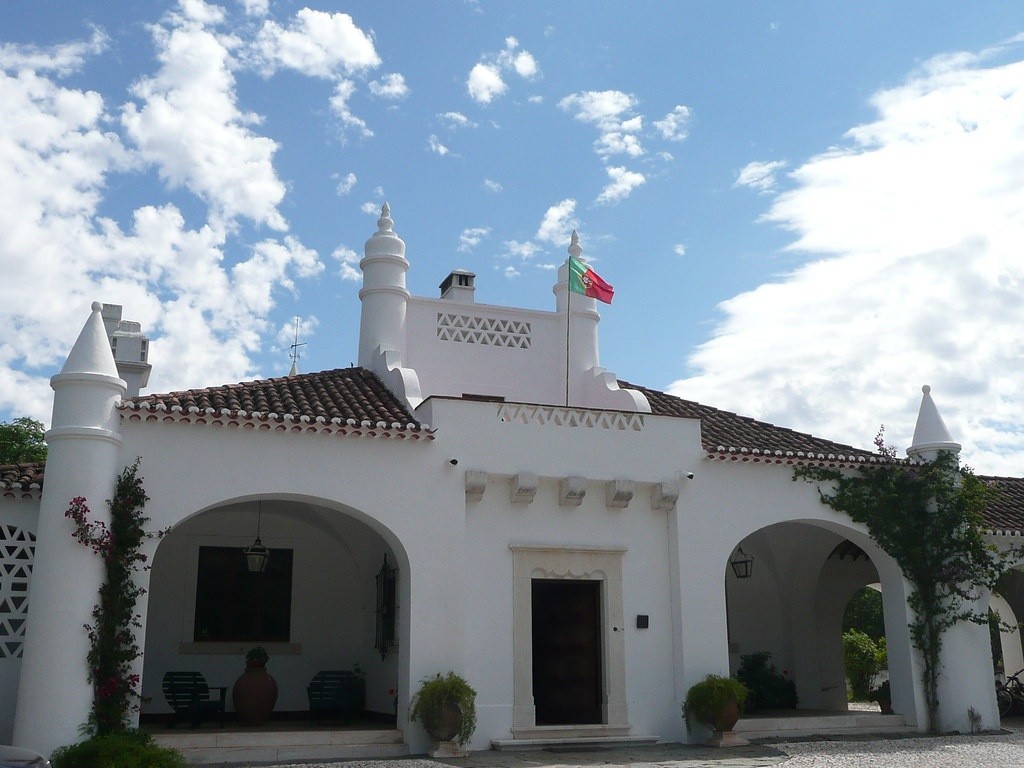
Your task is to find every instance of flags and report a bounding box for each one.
[568,257,613,305]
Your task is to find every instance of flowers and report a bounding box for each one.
[386,686,397,719]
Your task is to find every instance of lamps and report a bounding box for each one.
[242,500,271,576]
[729,543,755,578]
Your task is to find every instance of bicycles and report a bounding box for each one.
[995,669,1024,717]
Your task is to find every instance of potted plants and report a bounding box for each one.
[681,672,753,735]
[408,671,478,748]
[245,646,269,667]
[868,681,891,714]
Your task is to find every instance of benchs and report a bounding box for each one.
[306,670,366,725]
[162,671,229,731]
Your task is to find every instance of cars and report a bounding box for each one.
[0,745,52,768]
